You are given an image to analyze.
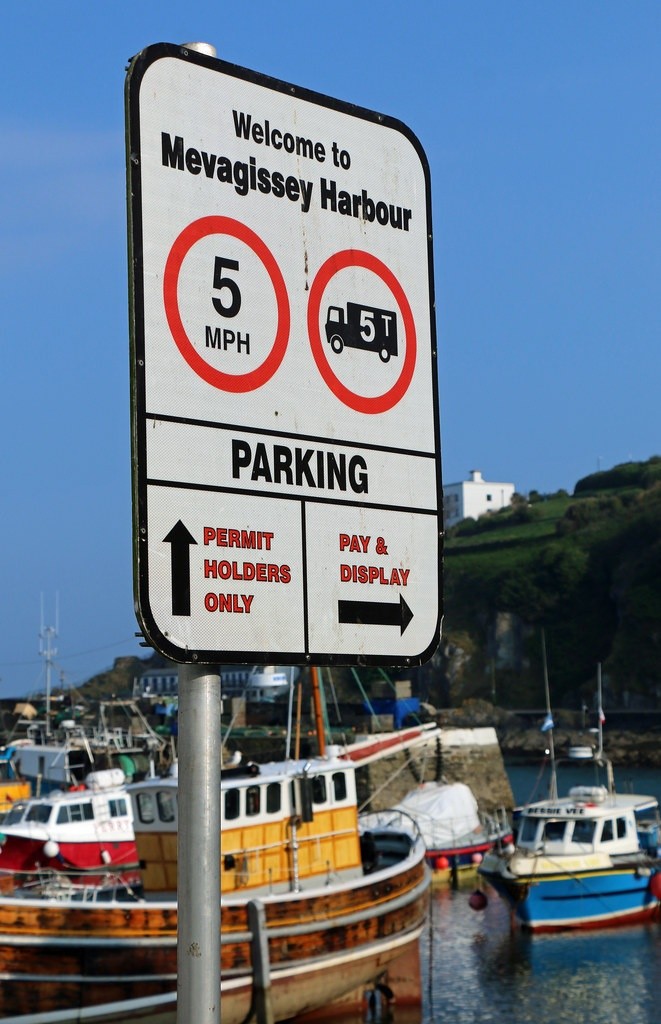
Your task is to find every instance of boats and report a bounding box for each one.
[0,591,660,1024]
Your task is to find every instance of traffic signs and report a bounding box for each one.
[122,42,444,664]
